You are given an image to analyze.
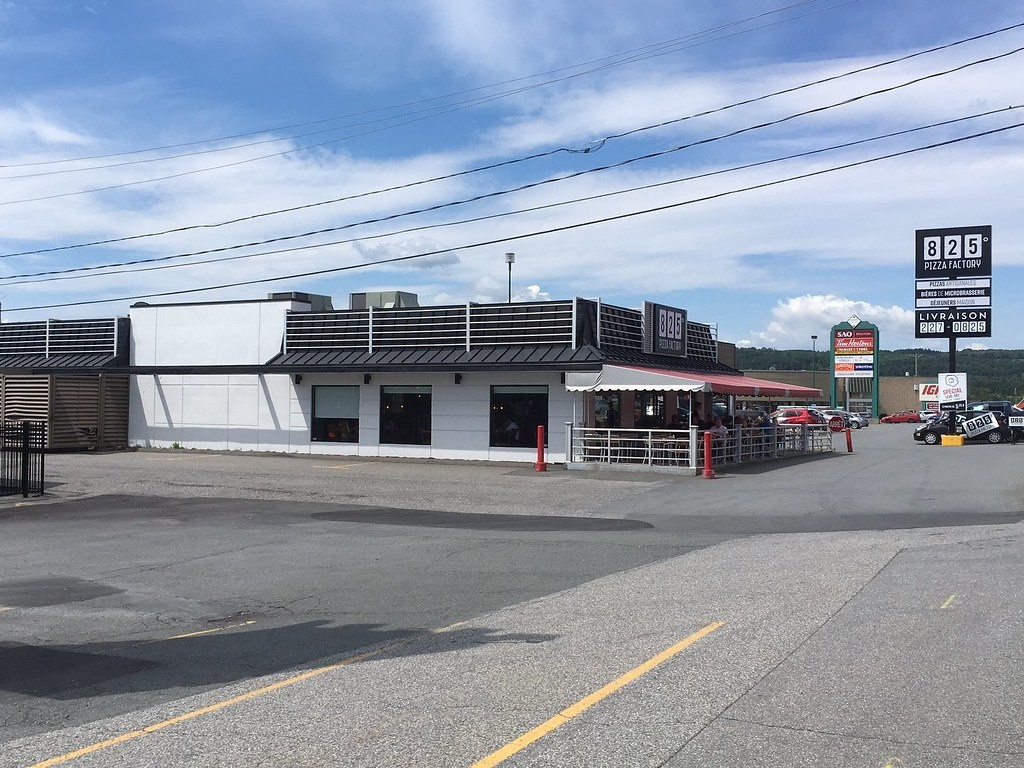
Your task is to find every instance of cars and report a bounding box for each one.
[1005,405,1024,442]
[649,407,689,422]
[820,409,869,431]
[881,411,920,423]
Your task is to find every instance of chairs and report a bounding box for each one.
[583,427,786,468]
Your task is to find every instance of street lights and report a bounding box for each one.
[505,252,516,304]
[812,336,817,405]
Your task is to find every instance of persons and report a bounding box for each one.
[705,412,781,457]
[691,415,706,436]
[606,401,619,427]
[695,401,702,418]
[667,414,682,430]
[709,417,729,461]
[505,418,520,440]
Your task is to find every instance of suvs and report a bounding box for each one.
[913,411,1013,444]
[916,411,938,423]
[767,408,819,433]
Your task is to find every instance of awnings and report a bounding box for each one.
[565,364,824,468]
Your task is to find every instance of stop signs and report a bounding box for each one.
[829,417,845,433]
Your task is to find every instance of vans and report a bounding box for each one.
[926,401,1013,428]
